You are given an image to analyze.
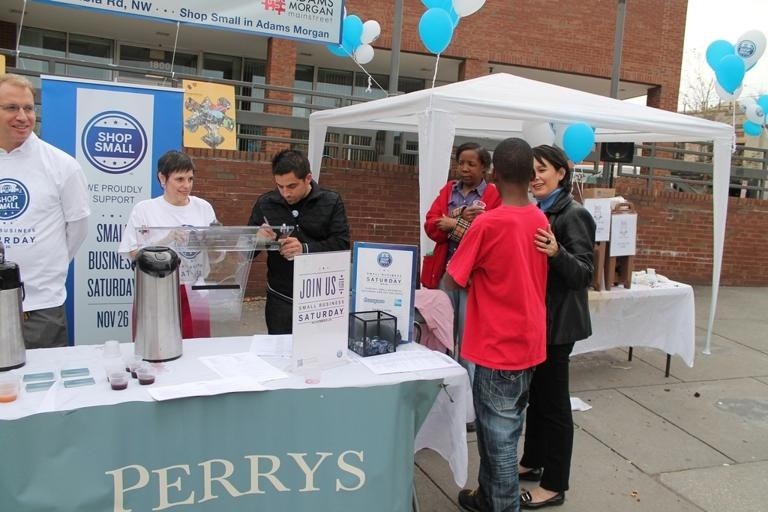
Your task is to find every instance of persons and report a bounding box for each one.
[239,149,352,336]
[420,141,502,438]
[0,70,87,350]
[438,136,551,512]
[117,149,222,343]
[518,142,596,508]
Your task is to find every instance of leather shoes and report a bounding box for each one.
[519,485,565,510]
[519,465,542,482]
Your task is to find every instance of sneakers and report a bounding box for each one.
[458,488,480,512]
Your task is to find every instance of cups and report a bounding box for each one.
[470,199,487,213]
[646,266,657,280]
[103,340,156,391]
[0,372,19,403]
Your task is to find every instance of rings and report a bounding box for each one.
[545,236,552,245]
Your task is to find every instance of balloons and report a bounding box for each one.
[558,118,594,165]
[705,25,768,136]
[326,3,380,68]
[453,1,486,16]
[419,0,449,9]
[418,7,451,57]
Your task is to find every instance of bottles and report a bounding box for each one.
[0,255,27,373]
[130,244,185,364]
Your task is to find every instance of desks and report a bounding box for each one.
[0,333,468,512]
[568,271,694,379]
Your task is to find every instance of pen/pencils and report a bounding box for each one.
[263,216,276,243]
